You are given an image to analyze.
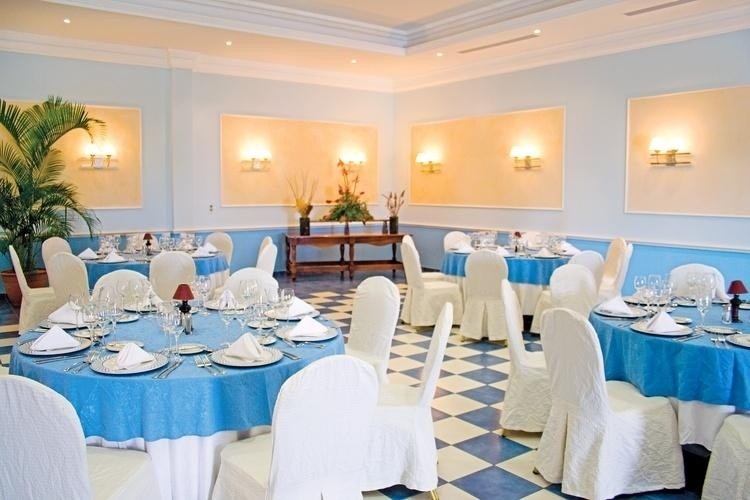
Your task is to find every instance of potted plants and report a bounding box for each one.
[381,189,407,234]
[0,93,112,310]
[319,157,373,236]
[282,167,319,236]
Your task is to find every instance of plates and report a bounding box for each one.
[106,341,144,351]
[17,332,91,356]
[594,295,750,350]
[265,308,321,320]
[192,254,216,258]
[203,301,250,310]
[124,303,157,311]
[88,256,105,259]
[453,250,576,259]
[96,260,128,263]
[172,344,208,355]
[276,326,337,341]
[116,314,138,322]
[73,328,110,337]
[209,347,283,368]
[90,352,168,374]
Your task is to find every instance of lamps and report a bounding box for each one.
[509,145,542,170]
[414,153,443,175]
[239,147,270,173]
[80,140,121,168]
[339,147,367,173]
[649,138,691,167]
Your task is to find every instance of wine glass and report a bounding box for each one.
[471,229,568,253]
[633,273,716,335]
[69,275,294,362]
[98,235,203,256]
